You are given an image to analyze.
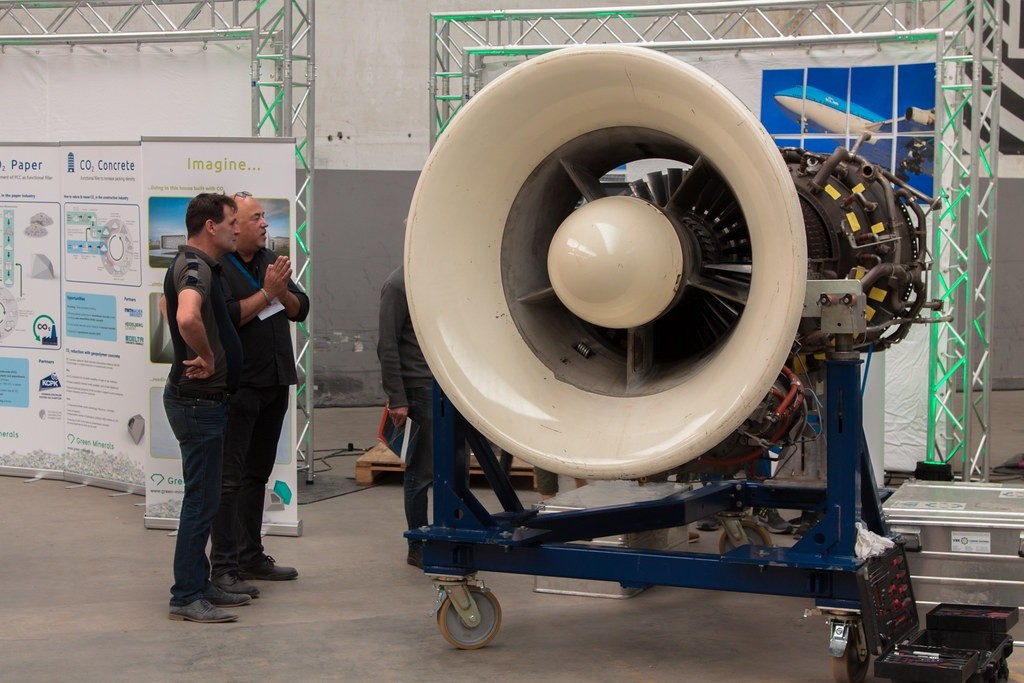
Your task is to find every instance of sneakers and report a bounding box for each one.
[695,514,720,532]
[750,507,794,534]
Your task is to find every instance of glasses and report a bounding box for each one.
[234,190,253,200]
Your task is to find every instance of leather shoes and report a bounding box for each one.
[168,598,240,623]
[200,580,253,608]
[237,554,300,582]
[209,570,261,599]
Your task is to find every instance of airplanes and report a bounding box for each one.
[770,84,936,147]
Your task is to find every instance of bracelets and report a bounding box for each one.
[260,288,271,305]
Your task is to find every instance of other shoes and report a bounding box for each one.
[687,531,700,544]
[791,510,819,540]
[406,546,425,571]
[786,516,802,528]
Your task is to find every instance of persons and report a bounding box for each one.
[377,265,431,569]
[210,193,298,597]
[688,446,793,542]
[170,193,251,625]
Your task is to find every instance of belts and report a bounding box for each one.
[165,385,231,401]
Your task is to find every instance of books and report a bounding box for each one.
[377,398,421,466]
